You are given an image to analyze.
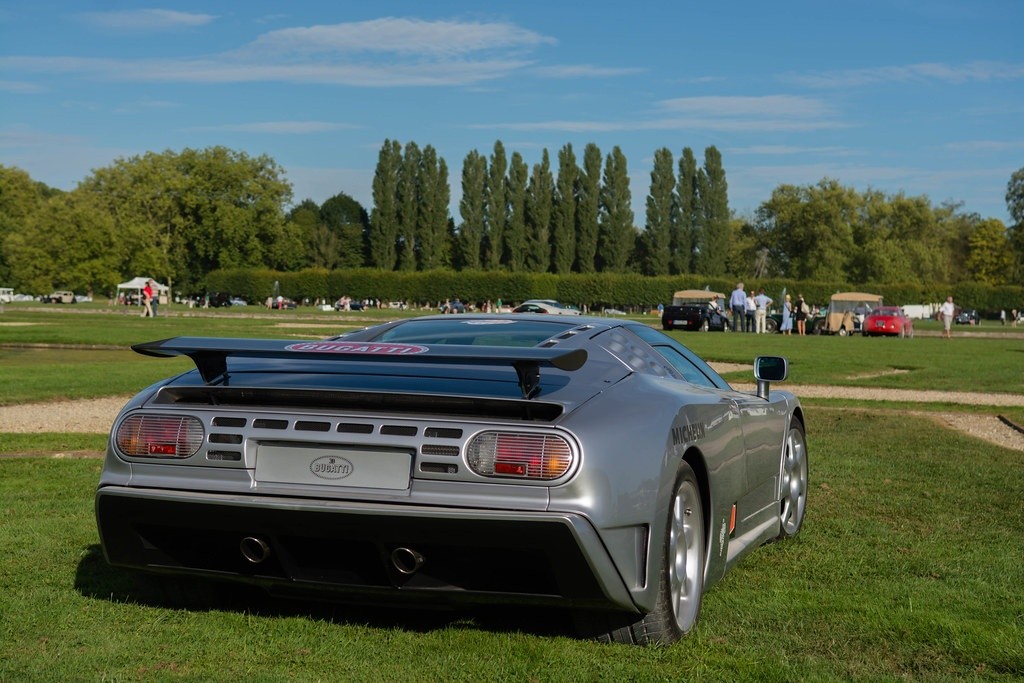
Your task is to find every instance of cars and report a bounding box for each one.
[861,307,916,339]
[40,291,79,305]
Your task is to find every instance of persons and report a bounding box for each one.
[966,308,1024,328]
[936,295,955,340]
[115,275,903,338]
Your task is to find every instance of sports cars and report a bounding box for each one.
[93,308,809,649]
[513,299,583,315]
[661,302,731,332]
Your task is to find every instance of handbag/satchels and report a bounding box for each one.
[789,311,794,318]
[801,301,809,314]
